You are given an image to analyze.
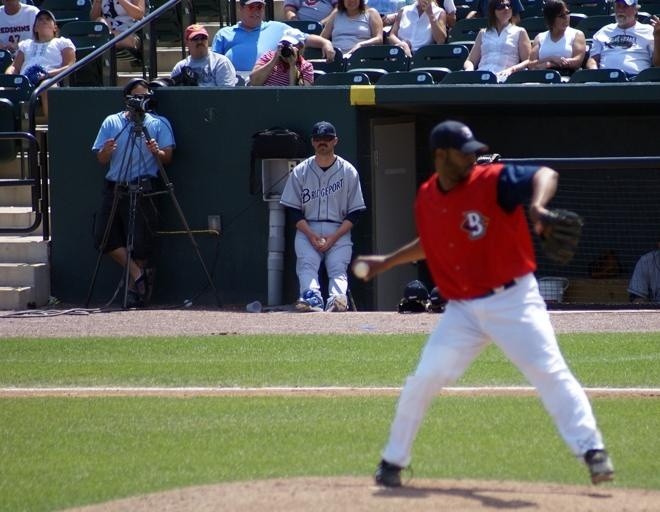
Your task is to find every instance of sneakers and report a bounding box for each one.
[584,449,614,483]
[122,265,152,308]
[325,295,347,312]
[376,460,405,486]
[296,289,324,312]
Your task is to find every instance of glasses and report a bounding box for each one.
[495,3,512,9]
[558,11,571,17]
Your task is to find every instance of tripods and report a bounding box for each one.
[85,126,224,311]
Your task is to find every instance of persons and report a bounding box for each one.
[5,10,76,119]
[626,250,660,309]
[1,1,40,56]
[246,28,314,87]
[91,77,179,314]
[168,25,238,87]
[350,118,618,492]
[276,122,365,312]
[90,0,145,48]
[284,0,660,84]
[210,0,336,70]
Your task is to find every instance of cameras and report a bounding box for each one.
[124,93,155,125]
[281,42,293,57]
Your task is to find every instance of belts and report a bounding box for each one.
[476,281,516,298]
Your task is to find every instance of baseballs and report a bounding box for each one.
[316,236,326,249]
[353,261,371,279]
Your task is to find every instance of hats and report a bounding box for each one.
[312,121,336,136]
[184,25,208,39]
[36,10,56,22]
[429,120,489,155]
[279,29,305,45]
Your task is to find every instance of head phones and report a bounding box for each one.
[124,77,149,95]
